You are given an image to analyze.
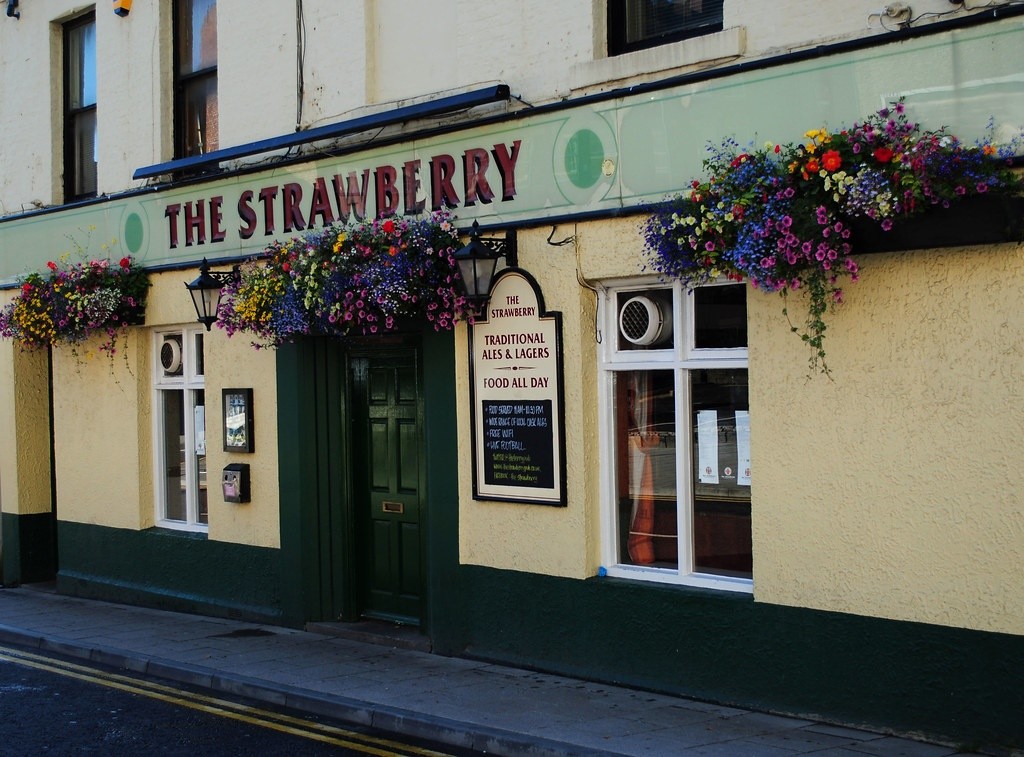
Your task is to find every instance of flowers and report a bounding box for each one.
[218,206,485,350]
[0,223,151,390]
[641,94,1024,382]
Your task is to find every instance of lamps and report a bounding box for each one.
[183,256,243,331]
[454,218,518,312]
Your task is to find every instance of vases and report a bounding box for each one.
[850,192,1024,254]
[100,304,145,327]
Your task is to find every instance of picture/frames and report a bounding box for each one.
[221,387,255,453]
